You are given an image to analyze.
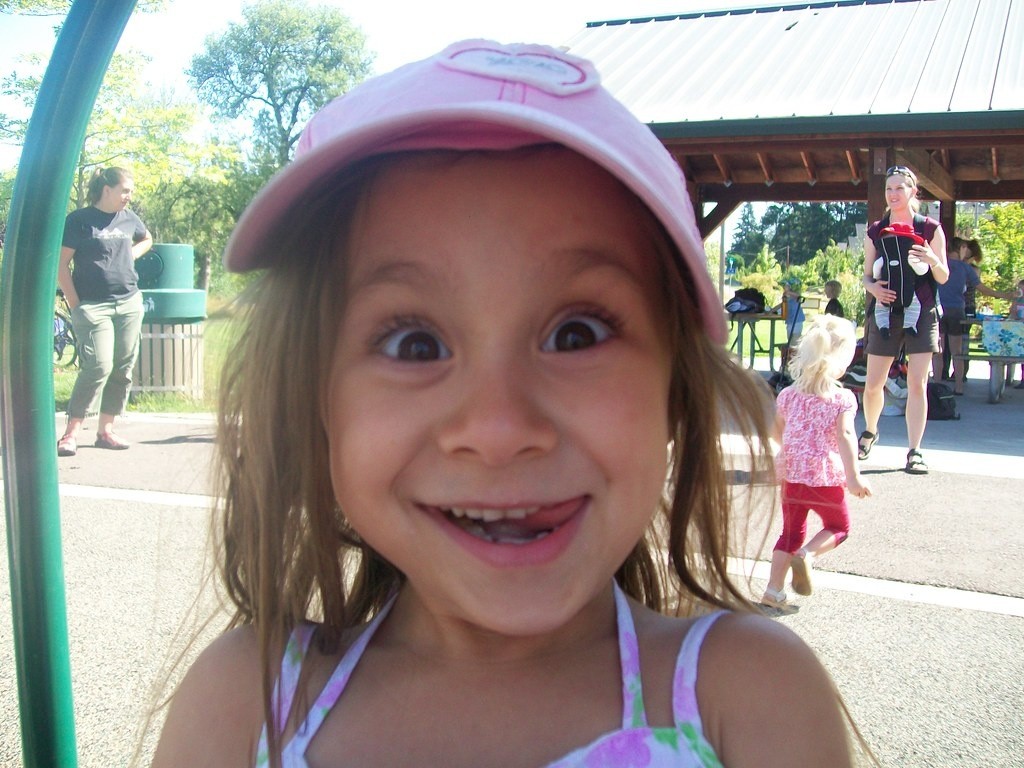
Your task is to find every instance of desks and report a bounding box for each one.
[725,314,784,373]
[961,320,1023,324]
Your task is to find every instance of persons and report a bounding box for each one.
[152,38,853,768]
[932,238,1019,395]
[770,277,806,344]
[1014,279,1024,389]
[857,166,949,474]
[825,280,844,317]
[760,313,872,613]
[54,169,152,457]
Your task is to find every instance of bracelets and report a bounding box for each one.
[932,262,939,268]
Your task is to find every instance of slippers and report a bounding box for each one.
[953,389,963,395]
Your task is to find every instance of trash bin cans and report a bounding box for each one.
[128,244,208,407]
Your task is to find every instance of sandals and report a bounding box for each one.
[791,548,813,596]
[761,589,790,610]
[857,431,880,460]
[906,448,928,474]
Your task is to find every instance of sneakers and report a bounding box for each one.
[58,433,77,455]
[95,432,130,450]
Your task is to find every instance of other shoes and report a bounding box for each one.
[1014,382,1024,389]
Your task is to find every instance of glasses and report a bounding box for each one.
[887,166,915,185]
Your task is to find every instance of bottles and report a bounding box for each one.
[781,295,788,320]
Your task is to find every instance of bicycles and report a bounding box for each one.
[52,289,78,368]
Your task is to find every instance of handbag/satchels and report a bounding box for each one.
[928,382,956,420]
[735,288,764,313]
[983,321,1024,356]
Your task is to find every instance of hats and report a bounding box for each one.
[779,277,801,296]
[879,222,924,245]
[227,39,729,348]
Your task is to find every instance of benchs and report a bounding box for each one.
[952,349,1024,404]
[773,344,784,373]
